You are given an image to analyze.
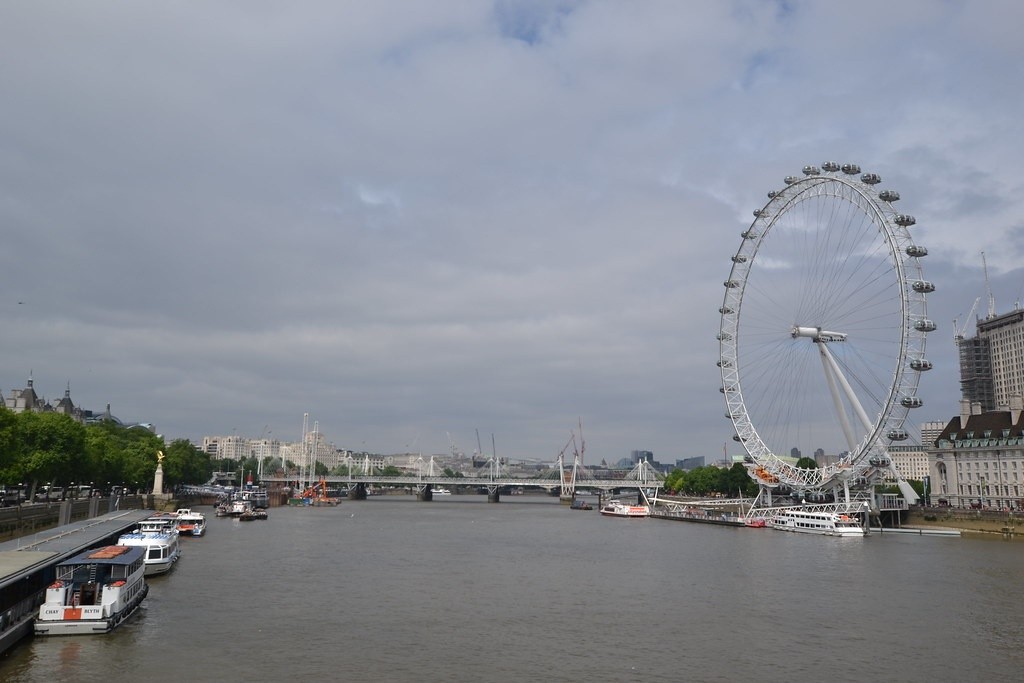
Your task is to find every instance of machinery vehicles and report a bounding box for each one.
[290,475,342,509]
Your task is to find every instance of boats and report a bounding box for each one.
[429,488,451,496]
[155,506,206,537]
[768,511,867,540]
[115,519,183,577]
[599,502,648,516]
[569,502,594,510]
[213,462,271,520]
[33,544,150,637]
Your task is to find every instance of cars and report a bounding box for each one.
[0,484,132,507]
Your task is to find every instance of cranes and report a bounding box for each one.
[557,416,586,465]
[491,431,498,460]
[475,427,484,458]
